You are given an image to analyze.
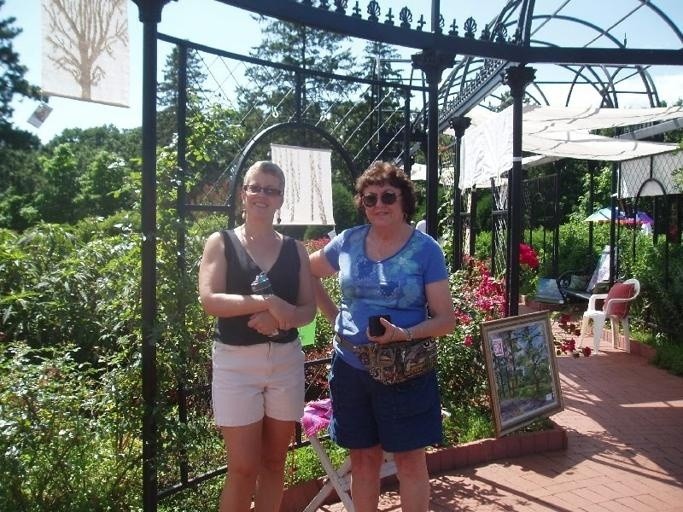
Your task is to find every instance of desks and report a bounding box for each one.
[301,409,450,512]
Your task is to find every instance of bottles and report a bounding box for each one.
[249,271,289,342]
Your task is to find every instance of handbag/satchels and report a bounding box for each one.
[357,336,435,387]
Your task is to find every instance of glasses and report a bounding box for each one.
[362,192,401,208]
[240,184,283,197]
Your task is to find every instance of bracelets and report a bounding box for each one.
[401,327,412,342]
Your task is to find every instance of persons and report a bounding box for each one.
[306,159,455,511]
[198,161,317,511]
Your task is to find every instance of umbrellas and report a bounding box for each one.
[614,212,654,225]
[584,206,627,222]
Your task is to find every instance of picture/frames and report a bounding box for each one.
[478,309,566,441]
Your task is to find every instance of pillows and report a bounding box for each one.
[602,283,635,320]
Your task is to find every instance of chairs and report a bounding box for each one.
[556,245,622,308]
[577,278,641,356]
